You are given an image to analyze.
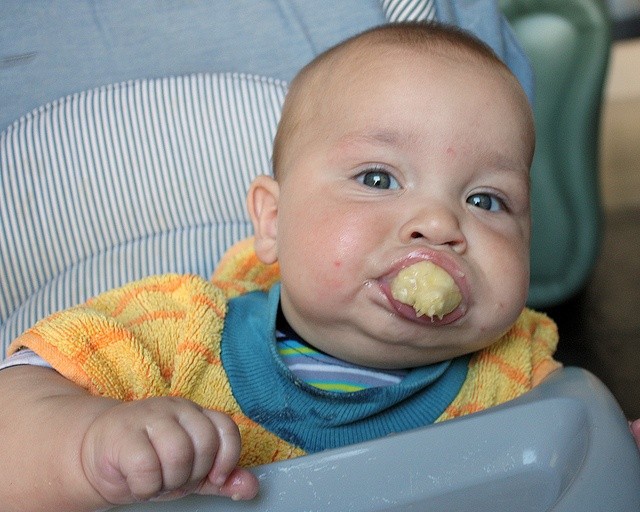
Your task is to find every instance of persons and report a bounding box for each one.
[0,17,640,511]
